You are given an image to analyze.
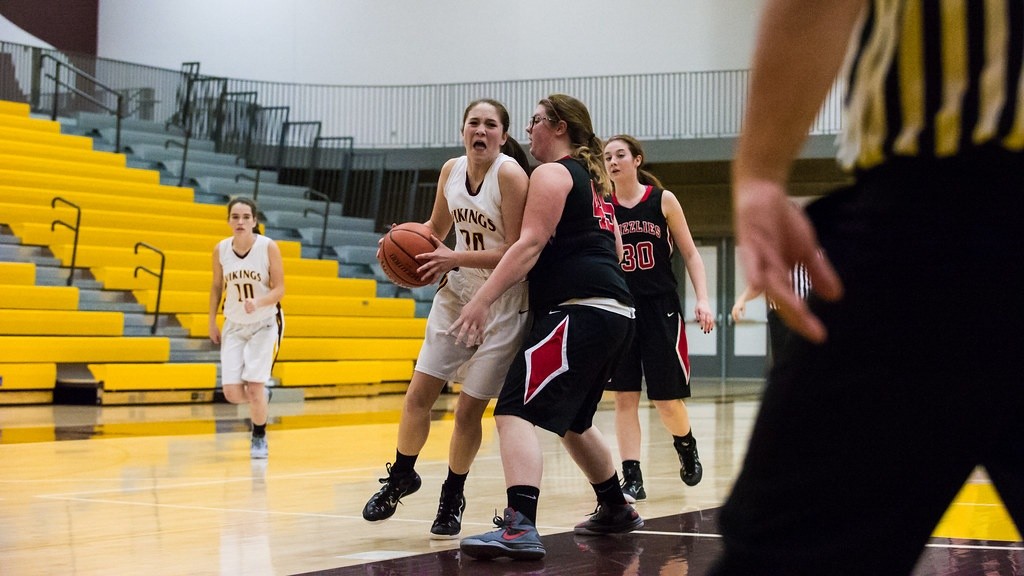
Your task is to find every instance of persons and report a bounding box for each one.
[361,99,529,540]
[707,1,1024,576]
[730,201,811,362]
[209,197,284,458]
[446,94,641,558]
[602,135,713,503]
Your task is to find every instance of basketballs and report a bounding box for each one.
[378,222,442,289]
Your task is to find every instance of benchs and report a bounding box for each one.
[0,94,460,407]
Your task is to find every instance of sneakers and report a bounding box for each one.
[574,498,645,534]
[264,388,273,402]
[673,437,702,486]
[251,435,268,458]
[363,462,421,521]
[619,477,646,502]
[460,507,546,560]
[430,484,466,539]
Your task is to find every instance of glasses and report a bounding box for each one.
[528,116,559,130]
